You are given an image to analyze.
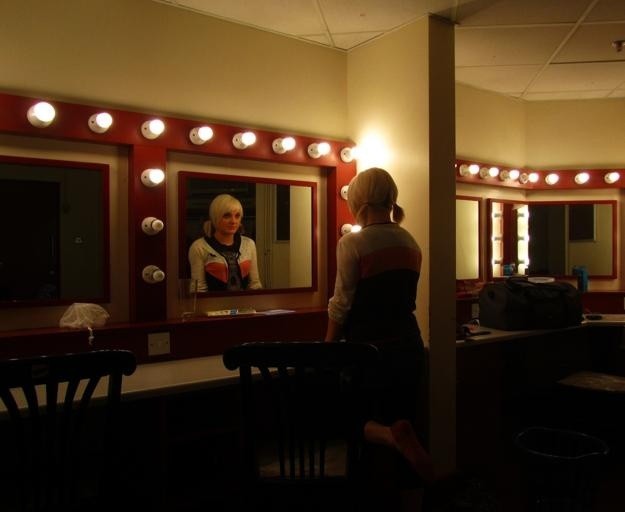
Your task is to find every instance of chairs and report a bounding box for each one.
[223,341,379,512]
[0,349,137,512]
[547,369,625,482]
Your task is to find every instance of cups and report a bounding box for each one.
[178,278,197,316]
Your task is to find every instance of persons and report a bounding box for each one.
[323,167,429,510]
[188,194,264,294]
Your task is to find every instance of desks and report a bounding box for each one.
[0,355,297,424]
[455,313,625,349]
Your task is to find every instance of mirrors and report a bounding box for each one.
[486,198,617,283]
[178,170,318,300]
[0,155,111,309]
[455,195,484,297]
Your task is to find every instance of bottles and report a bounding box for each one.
[577,266,588,293]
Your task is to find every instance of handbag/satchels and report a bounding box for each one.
[59,303,109,328]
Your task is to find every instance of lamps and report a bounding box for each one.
[459,164,620,185]
